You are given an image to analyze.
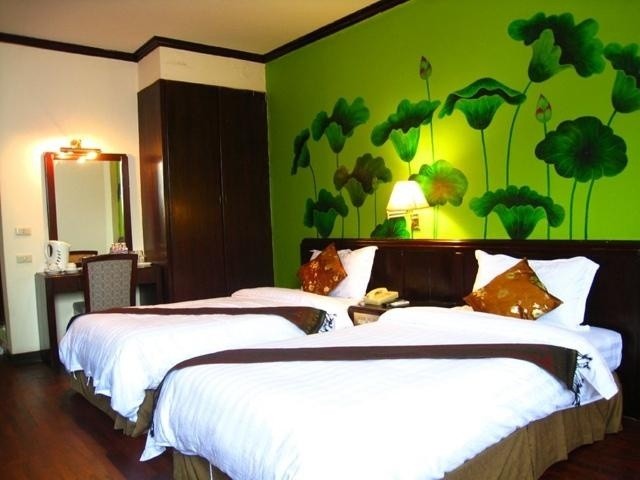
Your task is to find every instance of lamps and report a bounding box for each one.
[45,153,132,258]
[386,181,430,239]
[60,137,100,156]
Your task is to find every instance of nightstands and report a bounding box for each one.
[348,298,425,326]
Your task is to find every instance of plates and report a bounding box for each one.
[44,270,61,276]
[64,269,80,274]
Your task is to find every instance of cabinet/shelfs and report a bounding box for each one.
[136,80,271,301]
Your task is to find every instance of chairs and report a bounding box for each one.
[68,250,97,263]
[74,254,138,312]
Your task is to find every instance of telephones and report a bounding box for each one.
[366,287,399,305]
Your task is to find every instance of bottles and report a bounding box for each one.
[110,242,128,254]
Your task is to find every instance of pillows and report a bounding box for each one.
[460,257,562,321]
[300,246,377,302]
[473,249,600,327]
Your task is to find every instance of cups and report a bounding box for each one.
[48,263,59,271]
[66,262,77,269]
[129,251,144,263]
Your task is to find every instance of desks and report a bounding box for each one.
[35,265,164,373]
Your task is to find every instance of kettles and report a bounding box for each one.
[44,240,72,272]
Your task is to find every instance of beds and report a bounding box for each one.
[139,304,623,480]
[59,287,370,438]
